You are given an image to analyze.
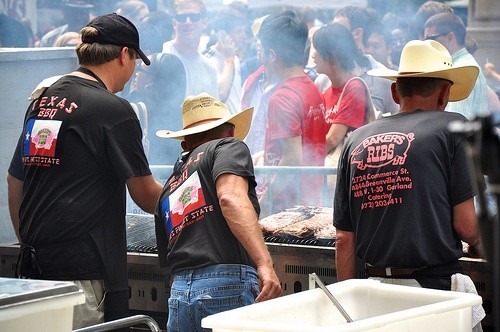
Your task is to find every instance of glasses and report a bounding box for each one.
[135,63,151,74]
[174,12,203,21]
[425,33,448,40]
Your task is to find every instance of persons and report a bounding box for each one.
[8,13,165,332]
[0,0,500,220]
[312,24,374,166]
[257,10,325,220]
[333,38,479,295]
[155,96,281,332]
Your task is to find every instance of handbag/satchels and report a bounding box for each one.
[324,77,383,189]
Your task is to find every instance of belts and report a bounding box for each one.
[368,267,425,277]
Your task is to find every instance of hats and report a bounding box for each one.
[63,0,94,8]
[155,92,254,141]
[367,40,480,102]
[82,13,151,65]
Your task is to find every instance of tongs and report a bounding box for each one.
[308,273,353,323]
